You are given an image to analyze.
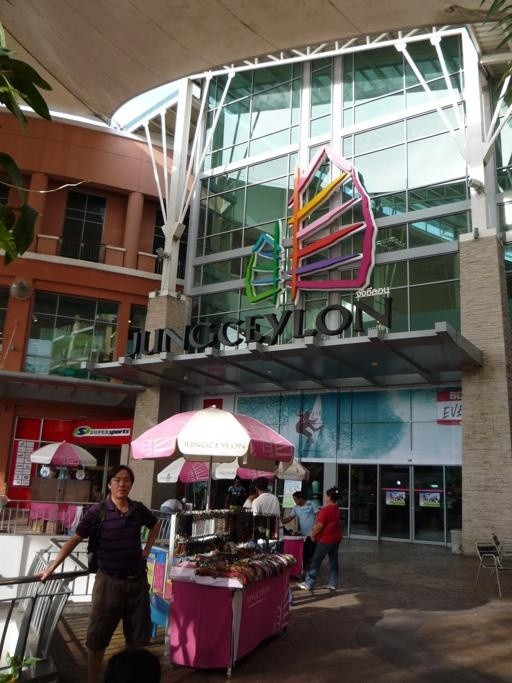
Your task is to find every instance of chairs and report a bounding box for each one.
[474,541,512,597]
[490,532,512,575]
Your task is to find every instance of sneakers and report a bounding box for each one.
[298,582,311,591]
[323,584,335,589]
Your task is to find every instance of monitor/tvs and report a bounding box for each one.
[385,490,406,507]
[417,492,442,508]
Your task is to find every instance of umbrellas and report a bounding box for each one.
[31,437,97,500]
[131,402,299,509]
[156,450,310,484]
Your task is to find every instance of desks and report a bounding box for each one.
[284,535,307,579]
[164,555,297,678]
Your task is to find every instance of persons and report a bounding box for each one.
[251,474,284,551]
[224,478,246,509]
[295,485,343,589]
[281,489,320,579]
[295,408,325,445]
[158,496,187,515]
[35,463,161,681]
[242,481,260,511]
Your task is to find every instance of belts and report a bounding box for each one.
[101,570,147,577]
